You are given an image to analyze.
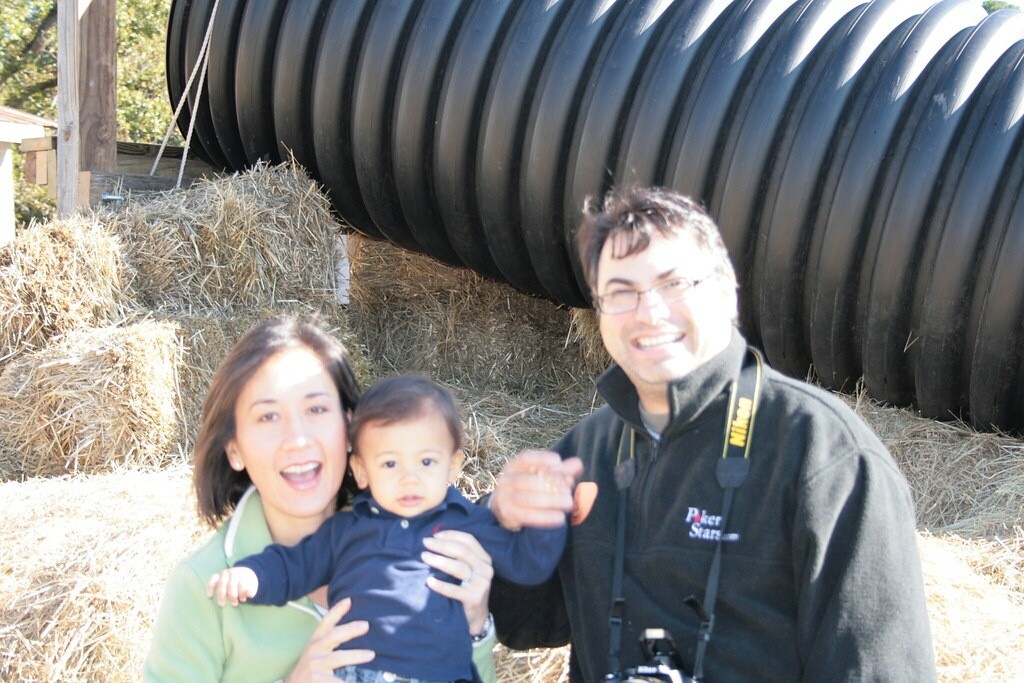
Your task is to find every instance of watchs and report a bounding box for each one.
[470,613,492,642]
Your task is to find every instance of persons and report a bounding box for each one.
[143,322,497,683]
[473,184,938,683]
[206,375,575,683]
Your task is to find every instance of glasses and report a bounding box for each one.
[597,273,714,315]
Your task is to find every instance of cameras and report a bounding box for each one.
[605,628,700,683]
